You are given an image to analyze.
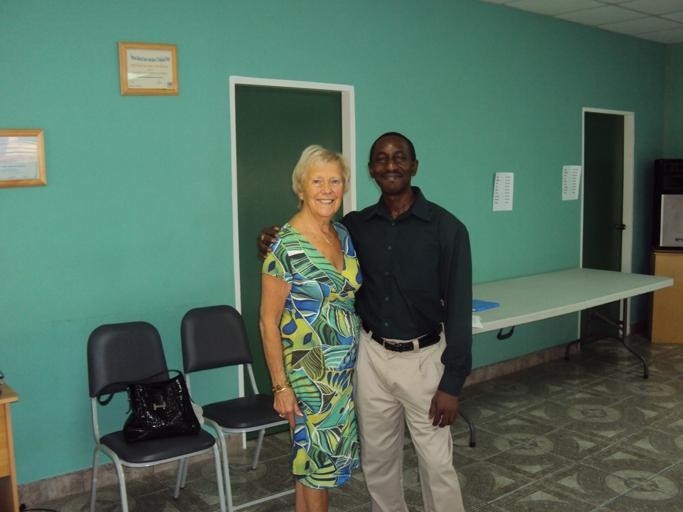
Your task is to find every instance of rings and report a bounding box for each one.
[278,413,285,418]
[259,234,267,241]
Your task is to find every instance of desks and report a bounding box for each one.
[442,264,674,449]
[0,377,24,512]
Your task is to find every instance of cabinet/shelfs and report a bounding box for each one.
[647,249,683,347]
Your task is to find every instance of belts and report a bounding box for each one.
[363,324,443,352]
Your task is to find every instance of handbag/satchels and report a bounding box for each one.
[124,375,200,442]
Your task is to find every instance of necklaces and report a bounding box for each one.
[315,220,339,246]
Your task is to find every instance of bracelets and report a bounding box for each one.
[270,383,292,393]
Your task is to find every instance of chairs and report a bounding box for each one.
[84,318,230,511]
[179,303,303,511]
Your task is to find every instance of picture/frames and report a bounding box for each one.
[118,39,180,97]
[0,127,48,190]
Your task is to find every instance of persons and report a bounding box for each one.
[255,129,474,512]
[254,140,368,512]
